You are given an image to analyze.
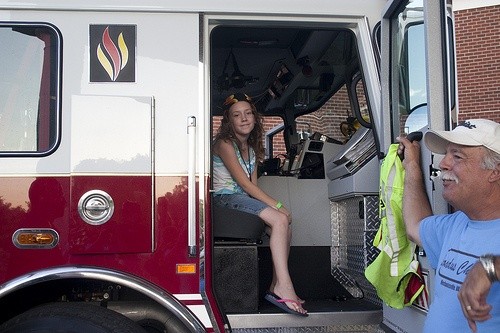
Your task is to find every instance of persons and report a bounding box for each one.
[213,93,309,317]
[396,119,500,333]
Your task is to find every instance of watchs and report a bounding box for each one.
[480,255,498,282]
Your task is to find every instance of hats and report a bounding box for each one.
[424,118,500,154]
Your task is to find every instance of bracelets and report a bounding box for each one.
[275,202,282,208]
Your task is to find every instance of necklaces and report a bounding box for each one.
[238,147,251,181]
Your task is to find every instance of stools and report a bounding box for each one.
[213,206,266,240]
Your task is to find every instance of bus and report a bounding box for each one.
[0,0,460,333]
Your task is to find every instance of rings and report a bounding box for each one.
[465,306,472,310]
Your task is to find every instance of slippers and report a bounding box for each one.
[264,294,308,317]
